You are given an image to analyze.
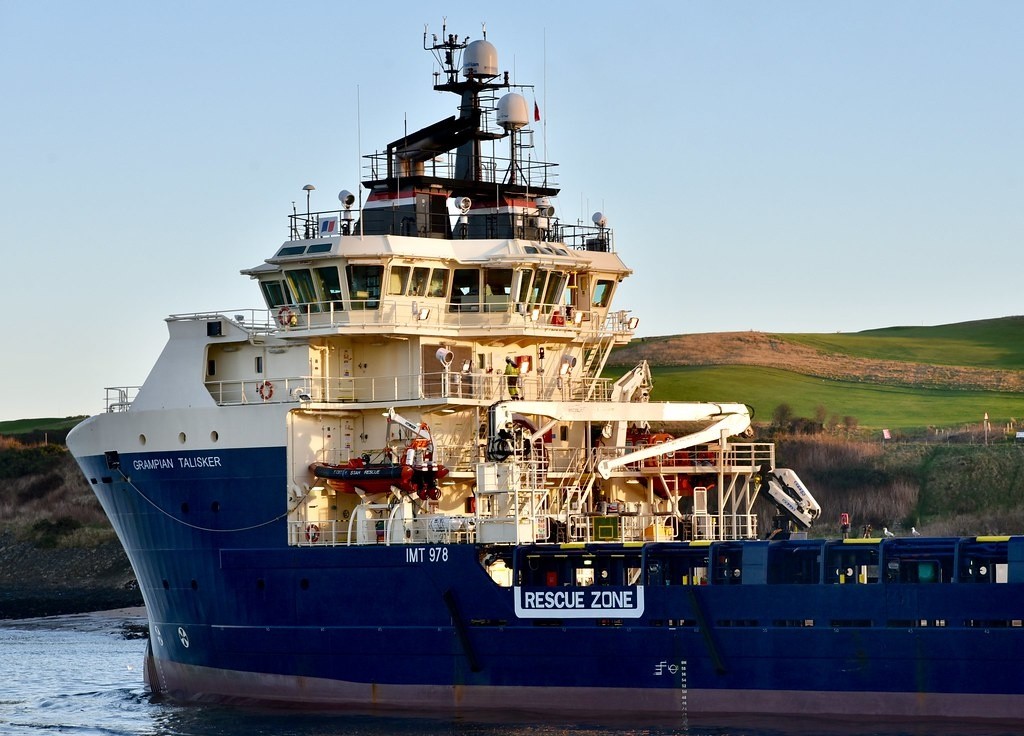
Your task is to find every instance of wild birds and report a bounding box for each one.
[911,526,923,537]
[883,527,896,538]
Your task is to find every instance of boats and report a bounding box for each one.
[763,468,821,520]
[66,19,1024,726]
[307,409,448,501]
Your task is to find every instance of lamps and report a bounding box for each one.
[542,206,554,217]
[455,198,472,223]
[531,309,541,322]
[562,355,576,368]
[339,190,355,219]
[628,318,639,329]
[572,311,582,323]
[435,348,454,372]
[418,308,430,320]
[593,212,607,226]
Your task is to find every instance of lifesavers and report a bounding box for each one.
[259,382,273,399]
[305,524,320,543]
[278,307,292,325]
